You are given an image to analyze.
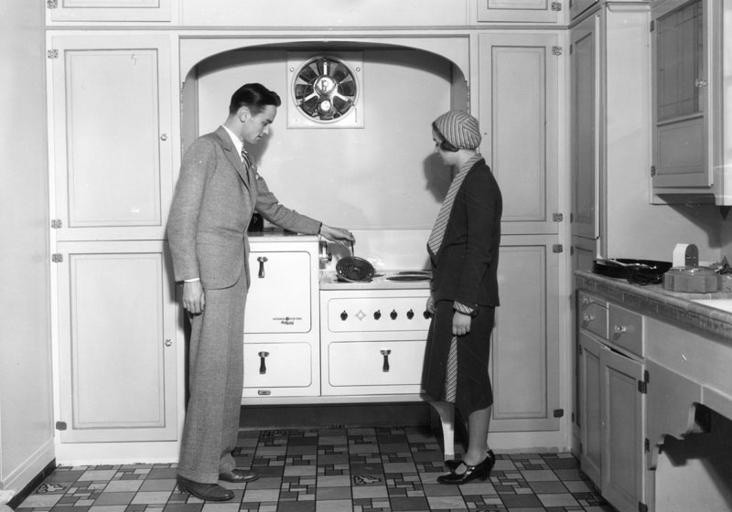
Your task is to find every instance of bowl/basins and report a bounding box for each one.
[336,256,374,283]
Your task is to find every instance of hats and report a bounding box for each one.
[434,110,481,149]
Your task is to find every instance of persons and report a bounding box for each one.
[165,82,356,501]
[420,110,504,484]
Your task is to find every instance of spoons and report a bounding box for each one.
[349,231,360,281]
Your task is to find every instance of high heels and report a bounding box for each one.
[437,449,495,484]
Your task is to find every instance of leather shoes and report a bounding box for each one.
[177,474,234,501]
[219,469,260,482]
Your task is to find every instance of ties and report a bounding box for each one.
[241,151,250,179]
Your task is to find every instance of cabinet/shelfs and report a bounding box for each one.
[466,0,572,459]
[576,291,648,511]
[575,2,731,264]
[45,1,185,468]
[242,241,432,401]
